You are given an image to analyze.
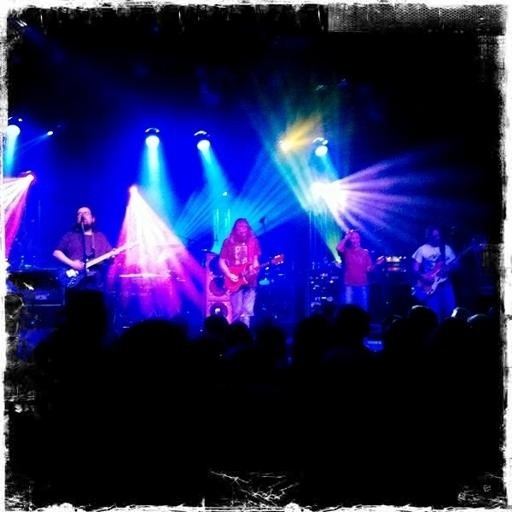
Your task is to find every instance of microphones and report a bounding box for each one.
[203,249,216,254]
[80,215,84,223]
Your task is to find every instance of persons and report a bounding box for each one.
[217,217,263,328]
[335,225,375,311]
[407,223,464,324]
[51,206,121,292]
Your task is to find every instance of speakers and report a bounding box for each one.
[206,254,232,325]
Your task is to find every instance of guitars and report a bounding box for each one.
[224,253,285,293]
[417,237,484,296]
[57,234,144,289]
